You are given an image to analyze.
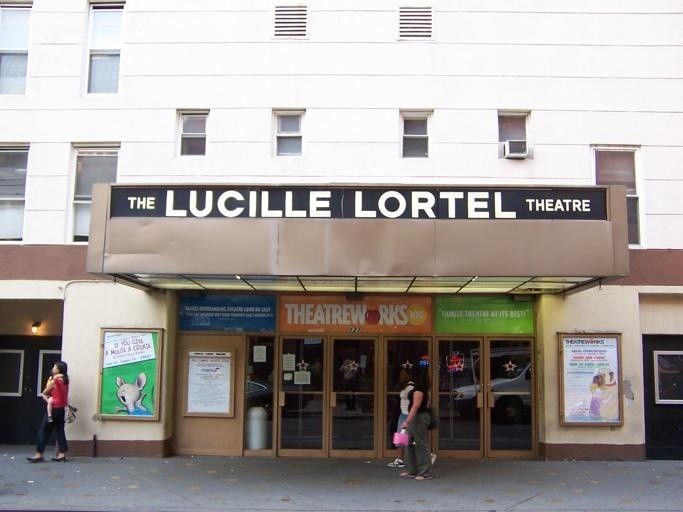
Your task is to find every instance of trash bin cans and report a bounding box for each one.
[244,406,269,450]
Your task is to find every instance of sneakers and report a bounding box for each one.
[27,456,43,462]
[51,456,66,462]
[401,472,432,480]
[388,458,406,468]
[430,453,437,465]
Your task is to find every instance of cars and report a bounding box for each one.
[449,356,531,423]
[244,378,307,418]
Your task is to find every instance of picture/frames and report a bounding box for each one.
[652,350,683,405]
[95,326,164,422]
[180,347,236,418]
[556,331,624,427]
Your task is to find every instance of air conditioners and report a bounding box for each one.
[503,139,528,159]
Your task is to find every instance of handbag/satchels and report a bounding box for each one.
[428,406,439,430]
[63,406,78,424]
[393,433,409,447]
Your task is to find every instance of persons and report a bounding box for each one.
[588,384,612,419]
[339,358,359,411]
[389,369,409,448]
[25,360,69,461]
[400,365,433,480]
[387,368,437,468]
[42,368,63,423]
